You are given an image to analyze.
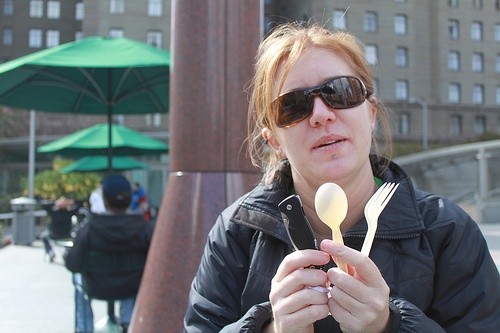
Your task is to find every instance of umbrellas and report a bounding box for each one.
[36,120,169,155]
[62,153,149,173]
[0,33,171,171]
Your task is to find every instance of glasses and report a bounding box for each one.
[270,75,368,129]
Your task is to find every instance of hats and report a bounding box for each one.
[101,175,132,199]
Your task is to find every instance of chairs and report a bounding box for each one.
[74,242,147,333]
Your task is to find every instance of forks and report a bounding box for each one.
[355,182,401,277]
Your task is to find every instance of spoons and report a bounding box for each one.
[315,182,349,275]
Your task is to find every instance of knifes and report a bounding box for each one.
[277,194,325,271]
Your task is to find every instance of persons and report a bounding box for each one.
[63,171,155,333]
[182,23,500,333]
[42,196,82,262]
[89,180,106,215]
